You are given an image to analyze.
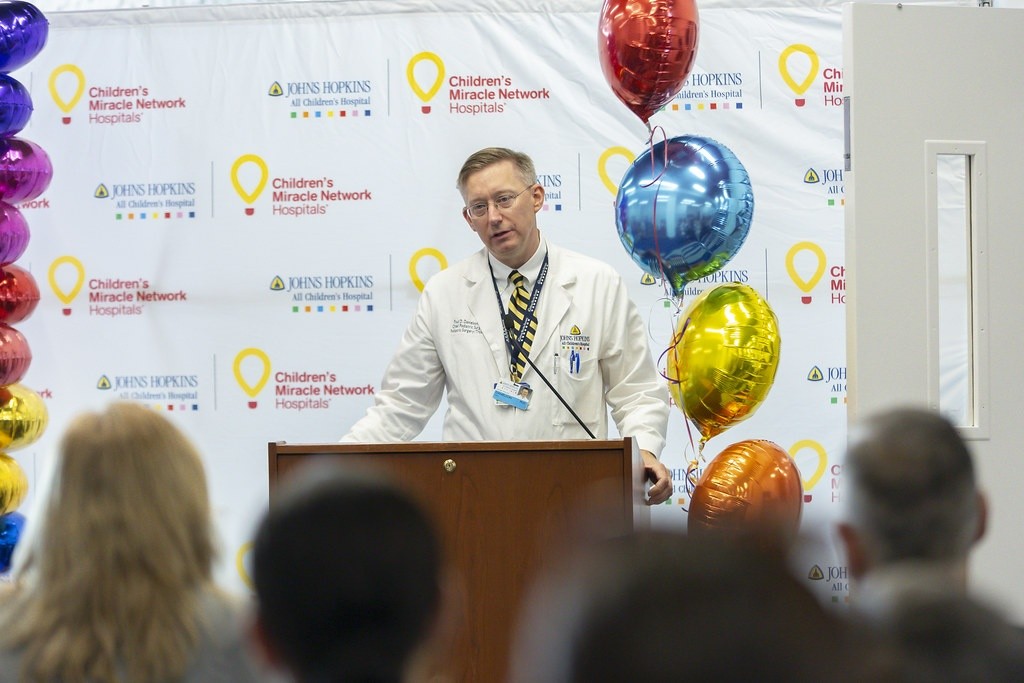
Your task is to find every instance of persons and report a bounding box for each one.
[248,477,441,683]
[517,388,529,401]
[538,533,831,683]
[342,147,672,507]
[1,405,256,683]
[789,408,1024,682]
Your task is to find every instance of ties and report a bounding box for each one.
[509,270,538,382]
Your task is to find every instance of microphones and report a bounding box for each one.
[504,314,597,439]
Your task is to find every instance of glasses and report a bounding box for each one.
[464,185,532,219]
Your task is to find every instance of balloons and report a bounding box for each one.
[599,0,700,126]
[667,281,780,445]
[0,0,50,575]
[689,439,800,540]
[616,135,753,294]
